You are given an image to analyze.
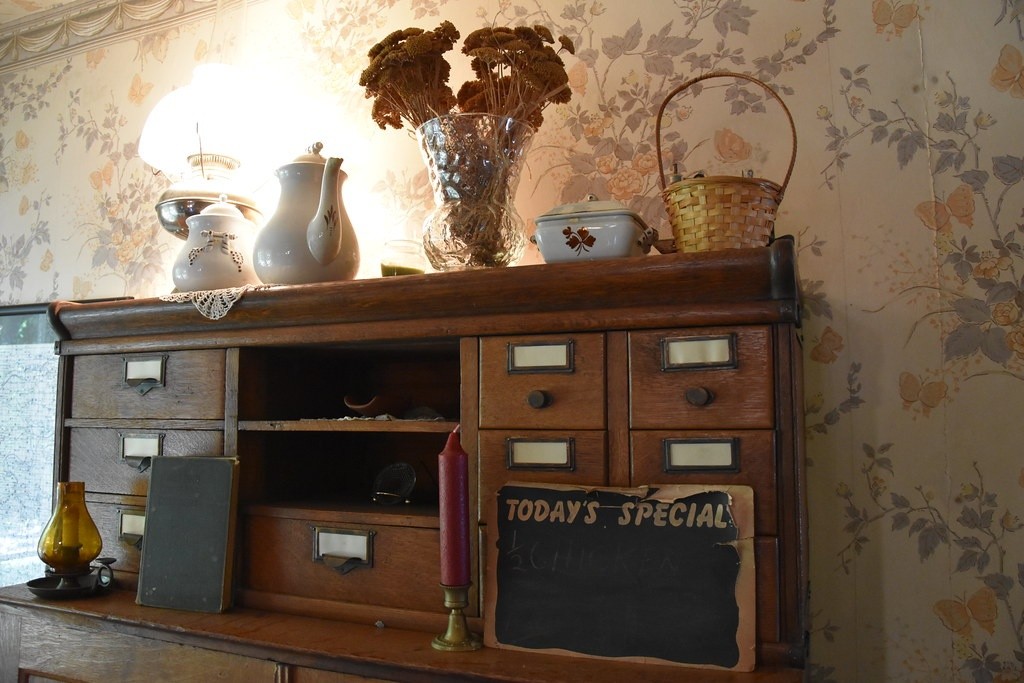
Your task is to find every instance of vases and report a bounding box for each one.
[421,113,535,269]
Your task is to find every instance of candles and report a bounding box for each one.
[437,424,471,585]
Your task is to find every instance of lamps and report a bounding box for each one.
[25,481,111,599]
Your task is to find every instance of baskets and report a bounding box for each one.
[654,70,801,252]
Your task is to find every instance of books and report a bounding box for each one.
[135,455,239,611]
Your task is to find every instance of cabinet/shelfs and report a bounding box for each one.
[0,233,811,683]
